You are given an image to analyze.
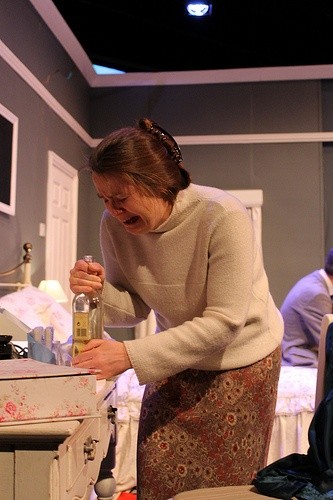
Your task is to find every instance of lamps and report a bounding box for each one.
[37,280,69,302]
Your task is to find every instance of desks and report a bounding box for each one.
[0,420,80,500]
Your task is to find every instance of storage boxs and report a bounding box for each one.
[0,357,103,427]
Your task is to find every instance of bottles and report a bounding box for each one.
[72,255,104,358]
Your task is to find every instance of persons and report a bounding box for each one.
[69,121,285,500]
[279,249,333,368]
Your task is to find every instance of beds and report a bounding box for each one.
[110,366,319,500]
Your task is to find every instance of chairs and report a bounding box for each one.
[168,313,333,500]
